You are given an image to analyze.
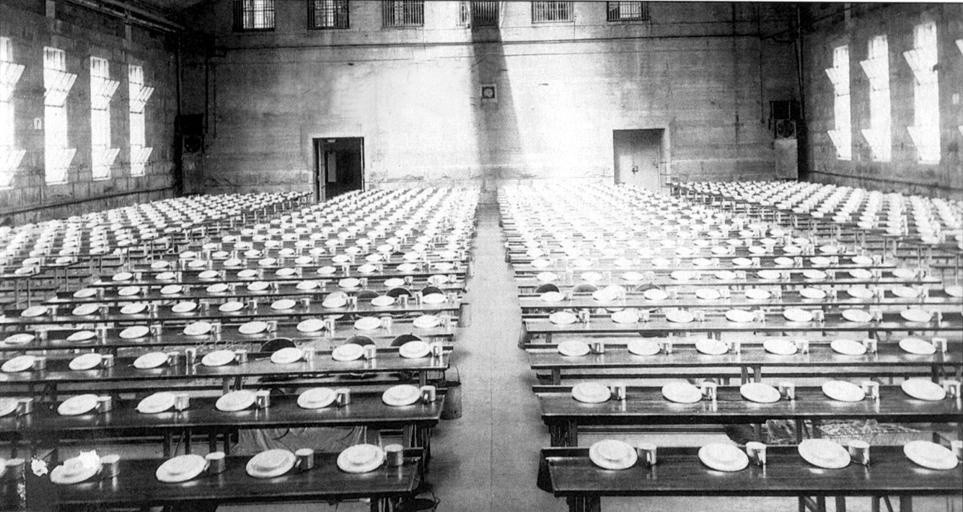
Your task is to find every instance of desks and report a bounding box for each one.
[0,181,963,512]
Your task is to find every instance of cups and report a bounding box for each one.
[863,382,880,401]
[206,451,227,473]
[779,384,795,399]
[702,383,717,402]
[385,443,406,469]
[943,380,960,401]
[94,396,113,414]
[744,440,767,464]
[15,398,34,415]
[101,454,122,478]
[847,439,873,466]
[335,388,350,407]
[5,457,25,480]
[255,390,271,409]
[421,386,437,403]
[610,383,626,400]
[638,440,659,465]
[174,394,190,411]
[296,447,316,472]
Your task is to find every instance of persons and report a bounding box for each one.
[324,140,341,200]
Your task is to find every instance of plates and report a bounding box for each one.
[740,383,781,403]
[821,380,865,404]
[1,397,16,416]
[154,454,208,484]
[215,390,254,412]
[796,439,853,469]
[57,394,95,415]
[586,440,637,472]
[338,443,385,473]
[570,382,611,404]
[2,247,460,368]
[901,380,946,403]
[296,388,335,410]
[247,445,296,478]
[695,442,751,474]
[138,392,176,413]
[662,383,702,403]
[899,438,958,472]
[382,385,420,406]
[532,256,963,366]
[51,456,98,484]
